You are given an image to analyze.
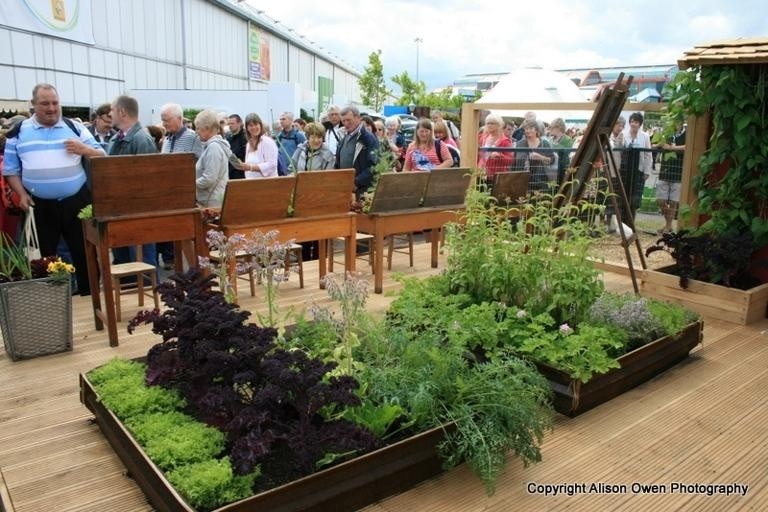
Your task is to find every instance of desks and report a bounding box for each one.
[478,169,536,256]
[76,151,210,351]
[343,165,476,297]
[201,166,360,306]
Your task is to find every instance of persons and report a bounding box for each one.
[0,84,687,298]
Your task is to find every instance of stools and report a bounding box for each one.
[326,231,376,280]
[256,241,307,292]
[368,230,415,274]
[207,245,258,307]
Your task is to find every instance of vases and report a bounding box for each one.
[0,273,74,362]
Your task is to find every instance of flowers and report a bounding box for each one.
[0,228,76,286]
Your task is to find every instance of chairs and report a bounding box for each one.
[107,242,159,323]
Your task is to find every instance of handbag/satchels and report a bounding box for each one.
[543,151,559,181]
[446,144,460,167]
[23,246,41,261]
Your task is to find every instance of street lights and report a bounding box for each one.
[413,37,423,85]
[409,97,415,115]
